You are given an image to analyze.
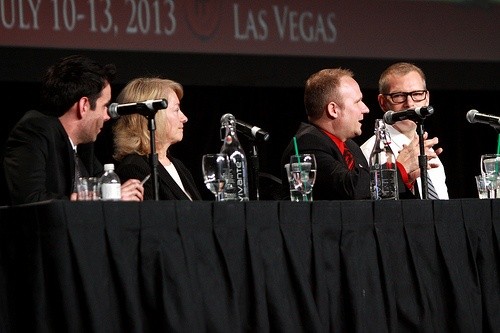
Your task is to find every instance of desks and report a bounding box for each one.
[0,198,500,333]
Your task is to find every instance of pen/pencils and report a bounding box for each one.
[140,173,151,187]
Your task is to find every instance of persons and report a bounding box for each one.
[282,68,442,200]
[112,77,217,201]
[0,55,144,203]
[360,62,449,199]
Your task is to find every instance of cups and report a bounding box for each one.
[284,162,312,202]
[475,175,489,199]
[77,177,98,200]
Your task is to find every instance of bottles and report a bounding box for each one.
[368,119,399,202]
[217,116,249,202]
[99,163,121,201]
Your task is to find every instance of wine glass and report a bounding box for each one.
[202,153,231,202]
[291,154,317,201]
[481,154,500,198]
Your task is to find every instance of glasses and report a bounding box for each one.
[383,90,429,102]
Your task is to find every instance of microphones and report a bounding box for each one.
[109,97,168,118]
[466,109,500,126]
[221,113,270,142]
[383,105,434,125]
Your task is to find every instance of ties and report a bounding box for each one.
[423,172,440,199]
